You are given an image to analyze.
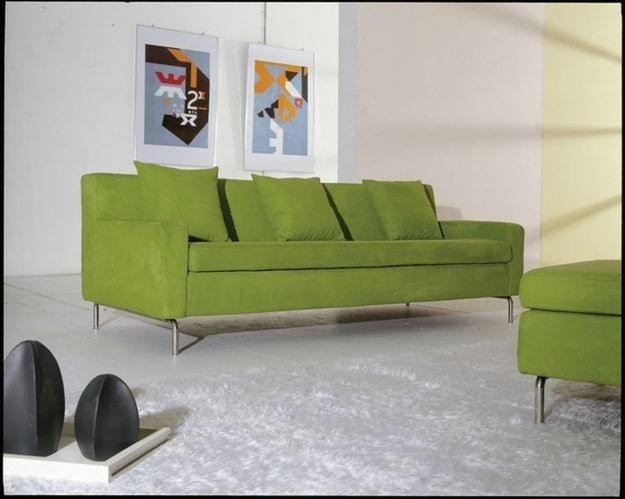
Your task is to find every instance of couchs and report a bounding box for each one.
[81,172,524,356]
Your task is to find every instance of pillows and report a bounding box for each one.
[251,173,348,241]
[133,160,232,242]
[362,179,444,241]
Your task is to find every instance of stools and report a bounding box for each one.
[517,260,621,423]
